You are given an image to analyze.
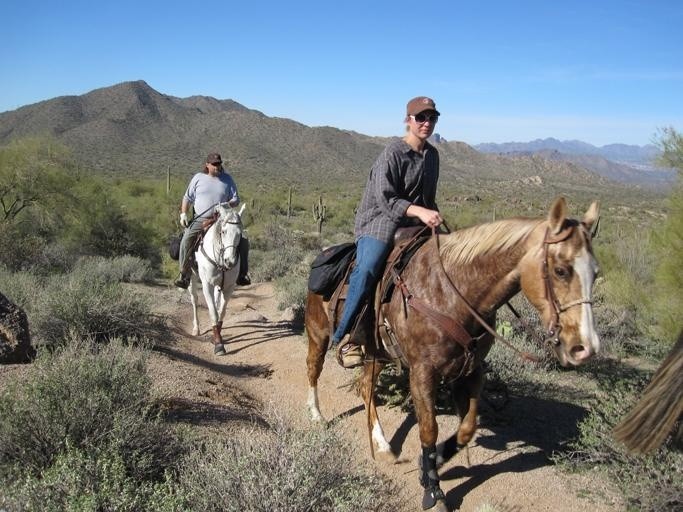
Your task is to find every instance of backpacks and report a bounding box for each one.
[307,242,356,293]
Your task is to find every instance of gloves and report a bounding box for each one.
[180,212,190,227]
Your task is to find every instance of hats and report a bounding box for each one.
[406,95,441,117]
[206,153,223,164]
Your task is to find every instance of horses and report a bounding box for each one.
[305,195,604,512]
[187,202,248,356]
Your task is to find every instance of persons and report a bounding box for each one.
[329,97,451,368]
[173,152,250,291]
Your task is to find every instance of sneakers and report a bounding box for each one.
[236,277,252,286]
[338,343,365,367]
[175,275,190,289]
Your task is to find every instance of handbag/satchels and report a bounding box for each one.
[168,233,183,260]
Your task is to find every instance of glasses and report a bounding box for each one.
[411,114,440,123]
